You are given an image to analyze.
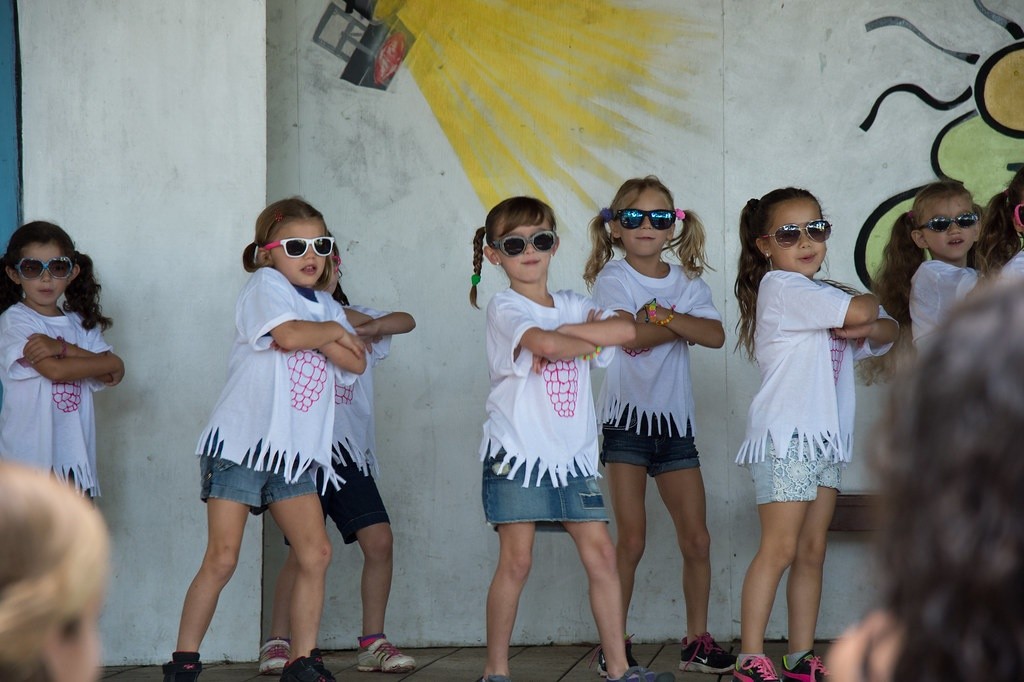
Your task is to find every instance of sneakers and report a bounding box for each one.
[606,666,675,682]
[781,650,830,682]
[679,632,737,673]
[589,634,638,677]
[279,648,338,682]
[355,637,416,673]
[259,637,291,675]
[476,674,511,682]
[162,651,202,682]
[733,655,780,682]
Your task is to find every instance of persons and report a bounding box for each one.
[255,231,417,672]
[0,220,125,509]
[466,196,678,682]
[0,455,112,682]
[727,185,900,682]
[827,163,1024,682]
[162,198,366,682]
[578,174,739,675]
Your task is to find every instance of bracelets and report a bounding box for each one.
[641,298,677,325]
[56,335,67,359]
[579,345,602,361]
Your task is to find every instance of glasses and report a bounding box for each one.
[266,236,335,257]
[613,209,676,230]
[919,212,978,232]
[16,257,72,280]
[493,231,555,257]
[331,255,341,273]
[762,220,831,250]
[1015,204,1024,226]
